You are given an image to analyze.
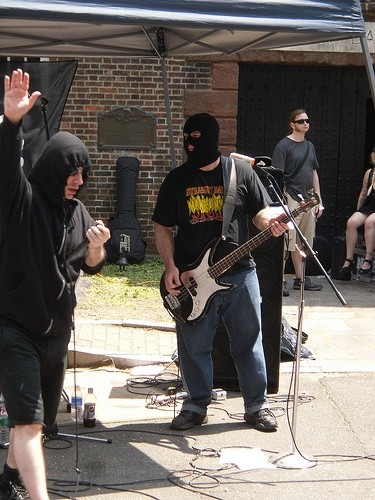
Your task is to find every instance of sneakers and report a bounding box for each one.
[244,408,277,430]
[293,277,322,290]
[283,281,289,295]
[0,475,30,500]
[172,410,208,429]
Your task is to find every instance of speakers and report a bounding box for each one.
[211,168,283,394]
[330,232,351,281]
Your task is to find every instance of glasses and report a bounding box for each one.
[293,119,310,124]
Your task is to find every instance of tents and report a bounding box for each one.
[0,0,375,167]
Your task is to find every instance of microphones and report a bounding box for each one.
[253,165,276,181]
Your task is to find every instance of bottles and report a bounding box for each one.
[83,388,97,428]
[71,385,83,421]
[0,392,10,445]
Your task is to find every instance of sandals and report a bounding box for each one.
[358,259,373,273]
[339,259,356,272]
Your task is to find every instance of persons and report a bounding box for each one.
[340,148,375,272]
[0,68,110,500]
[272,109,324,296]
[151,112,289,429]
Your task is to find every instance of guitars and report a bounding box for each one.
[159,187,325,327]
